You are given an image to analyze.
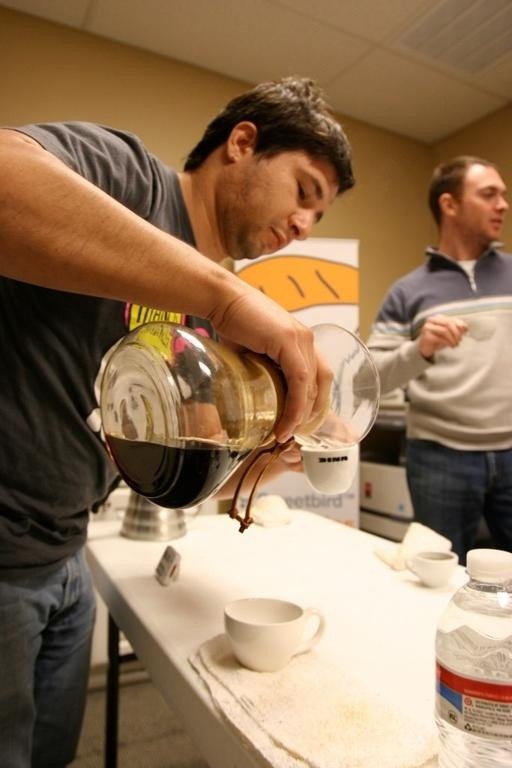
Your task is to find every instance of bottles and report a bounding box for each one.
[99,320,381,509]
[435,549,512,768]
[117,487,187,540]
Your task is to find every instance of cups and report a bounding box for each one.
[222,598,326,672]
[408,550,459,589]
[296,441,361,496]
[459,316,498,340]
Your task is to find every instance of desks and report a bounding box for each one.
[78,504,512,767]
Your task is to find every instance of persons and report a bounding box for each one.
[354,154,511,568]
[2,75,359,765]
